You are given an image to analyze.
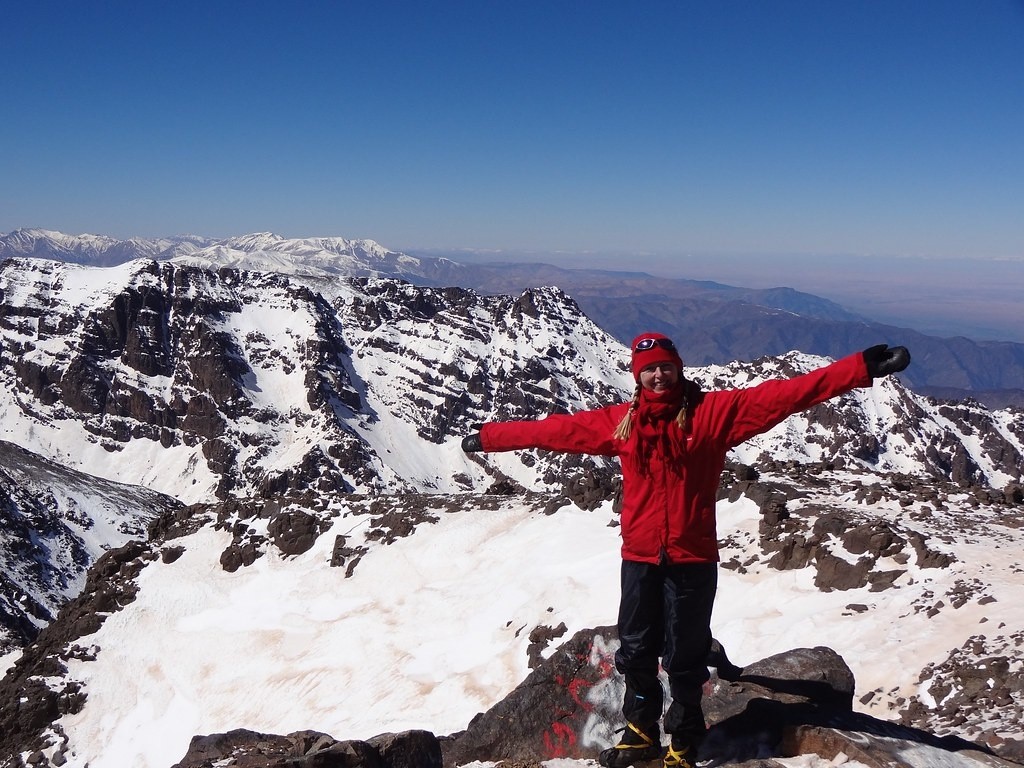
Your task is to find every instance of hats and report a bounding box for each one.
[630,332,683,384]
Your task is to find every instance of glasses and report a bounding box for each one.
[634,338,675,353]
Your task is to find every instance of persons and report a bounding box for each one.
[466,334,911,768]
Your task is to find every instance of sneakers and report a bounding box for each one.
[599,724,662,768]
[664,728,708,768]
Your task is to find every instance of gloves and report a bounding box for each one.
[461,423,484,452]
[862,344,911,379]
